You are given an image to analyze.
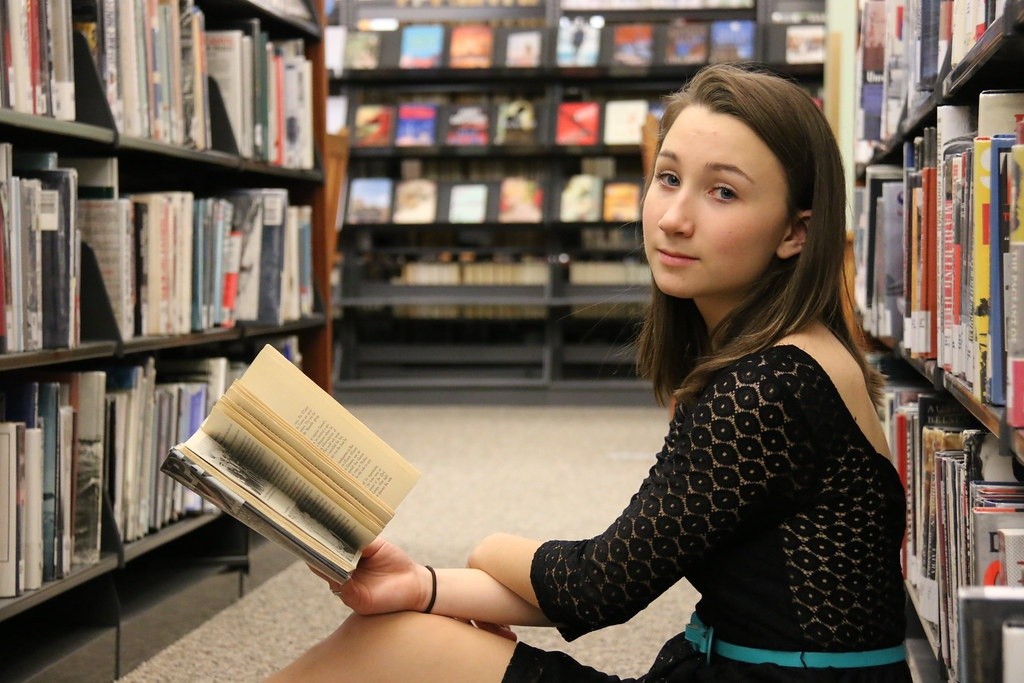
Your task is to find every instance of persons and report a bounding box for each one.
[257,62,916,682]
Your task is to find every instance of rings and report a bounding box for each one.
[332,589,341,596]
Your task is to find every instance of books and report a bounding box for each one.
[852,4,1024,682]
[349,13,826,319]
[1,0,319,599]
[161,343,422,587]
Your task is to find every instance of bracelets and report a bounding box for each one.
[423,565,437,615]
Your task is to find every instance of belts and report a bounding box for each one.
[684,611,905,668]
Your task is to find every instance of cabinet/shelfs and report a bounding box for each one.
[820,0,1024,683]
[327,1,827,390]
[1,1,334,683]
